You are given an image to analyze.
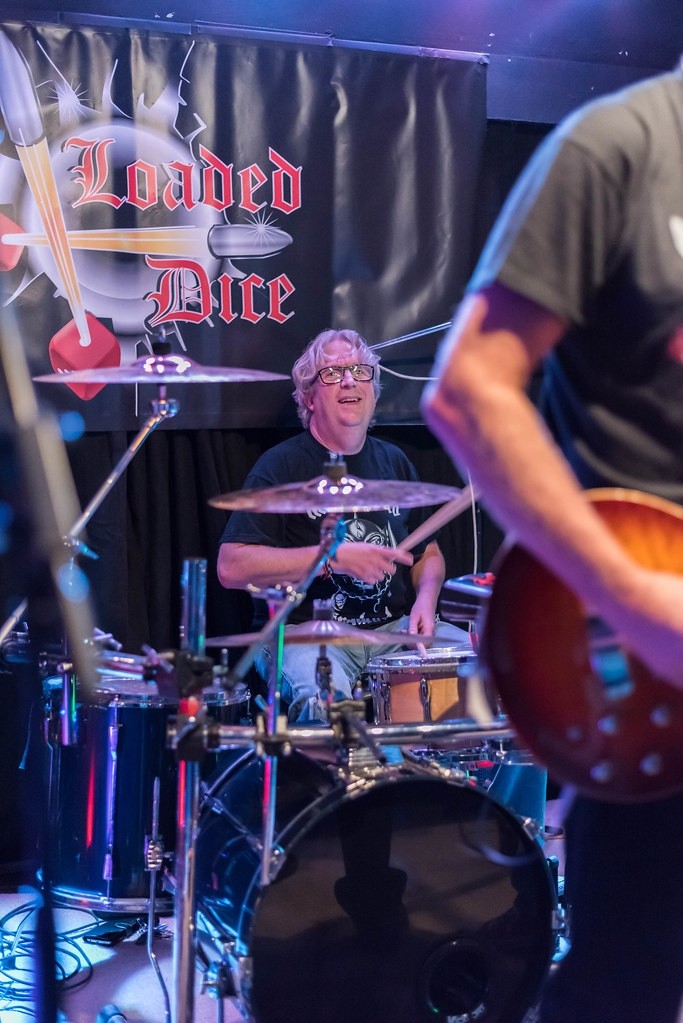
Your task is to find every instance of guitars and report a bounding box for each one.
[474,475,682,805]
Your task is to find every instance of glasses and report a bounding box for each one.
[304,364,375,390]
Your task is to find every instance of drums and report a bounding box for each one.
[26,650,561,1023]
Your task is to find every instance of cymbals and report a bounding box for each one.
[199,618,467,650]
[207,471,465,515]
[30,352,292,385]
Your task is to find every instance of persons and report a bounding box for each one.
[216,329,547,853]
[420,53,683,1023]
